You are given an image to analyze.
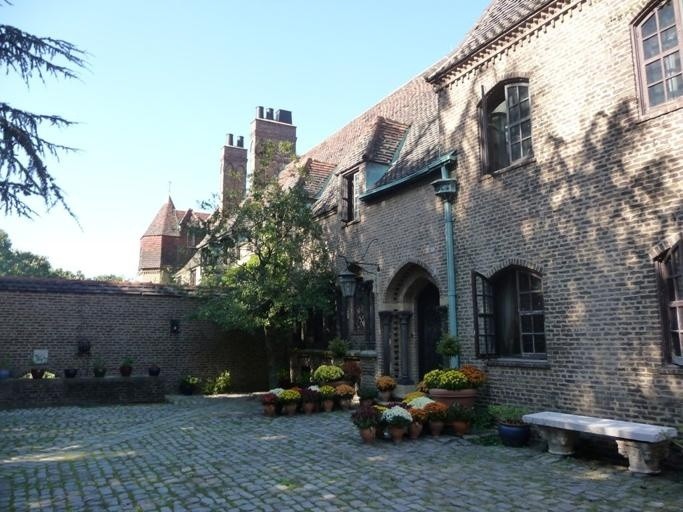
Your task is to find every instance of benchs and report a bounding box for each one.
[522,411,678,475]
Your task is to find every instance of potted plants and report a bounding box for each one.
[30,357,161,379]
[488,404,533,447]
[182,376,197,395]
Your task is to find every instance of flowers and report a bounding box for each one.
[261,365,483,424]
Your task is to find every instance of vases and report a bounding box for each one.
[360,421,466,445]
